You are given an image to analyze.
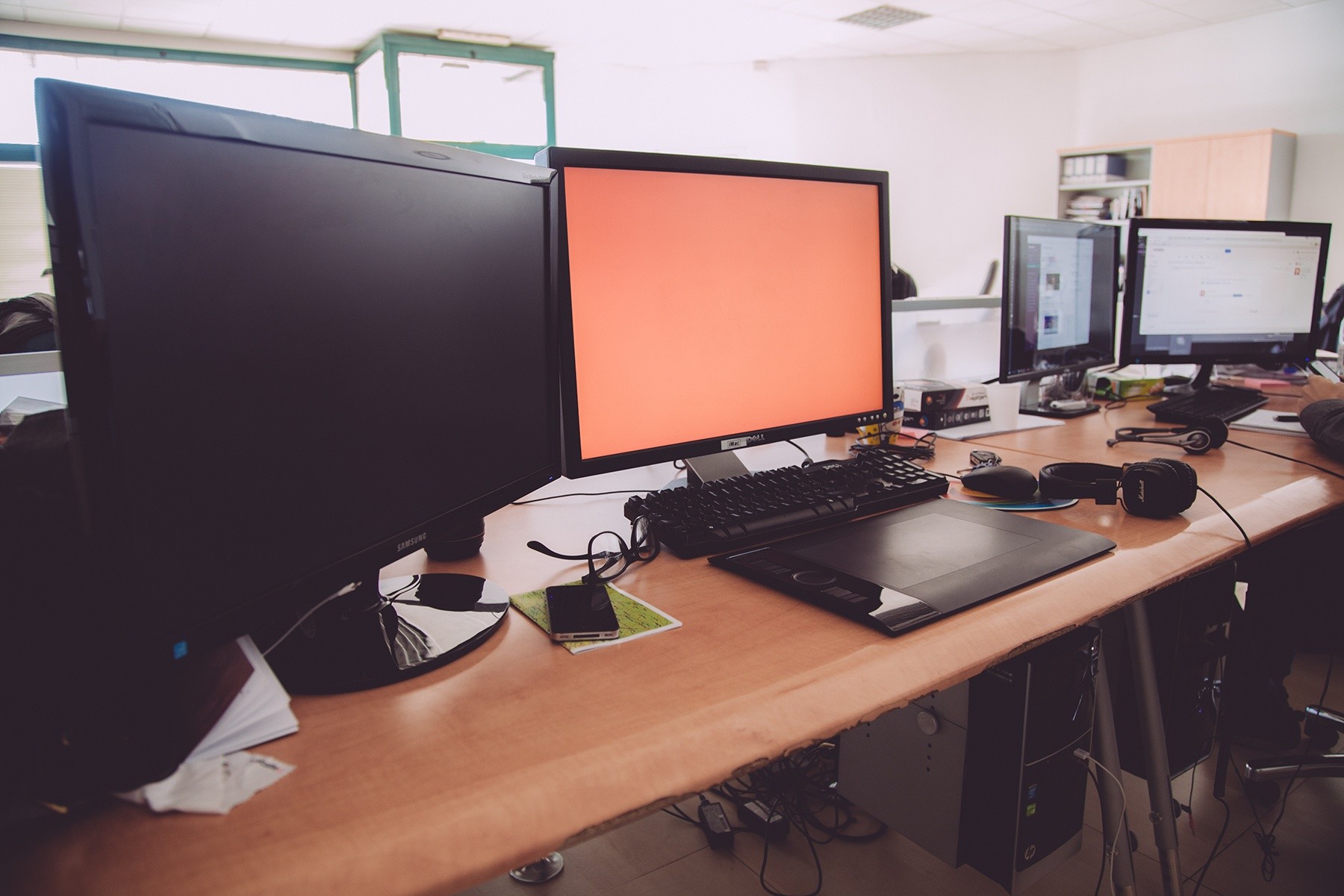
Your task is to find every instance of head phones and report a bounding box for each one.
[1038,456,1199,518]
[1113,413,1230,455]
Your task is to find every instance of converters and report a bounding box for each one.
[659,793,737,852]
[716,786,792,842]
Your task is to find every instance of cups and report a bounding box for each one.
[986,383,1020,427]
[856,381,905,444]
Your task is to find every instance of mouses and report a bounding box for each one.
[959,464,1038,499]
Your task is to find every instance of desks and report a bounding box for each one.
[0,368,1343,896]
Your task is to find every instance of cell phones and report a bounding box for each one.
[544,582,620,643]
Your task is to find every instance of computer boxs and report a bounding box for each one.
[836,622,1102,896]
[1089,558,1242,783]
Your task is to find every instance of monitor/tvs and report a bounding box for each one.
[995,214,1119,422]
[532,143,898,482]
[1116,217,1331,401]
[33,74,572,702]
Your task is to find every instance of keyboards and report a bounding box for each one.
[619,448,952,562]
[1145,387,1271,427]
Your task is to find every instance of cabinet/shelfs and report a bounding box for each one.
[1149,128,1299,219]
[1055,143,1149,226]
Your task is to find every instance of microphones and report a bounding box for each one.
[1105,438,1199,447]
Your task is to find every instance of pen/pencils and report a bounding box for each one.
[1272,414,1301,423]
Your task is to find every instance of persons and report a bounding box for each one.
[1297,374,1344,464]
